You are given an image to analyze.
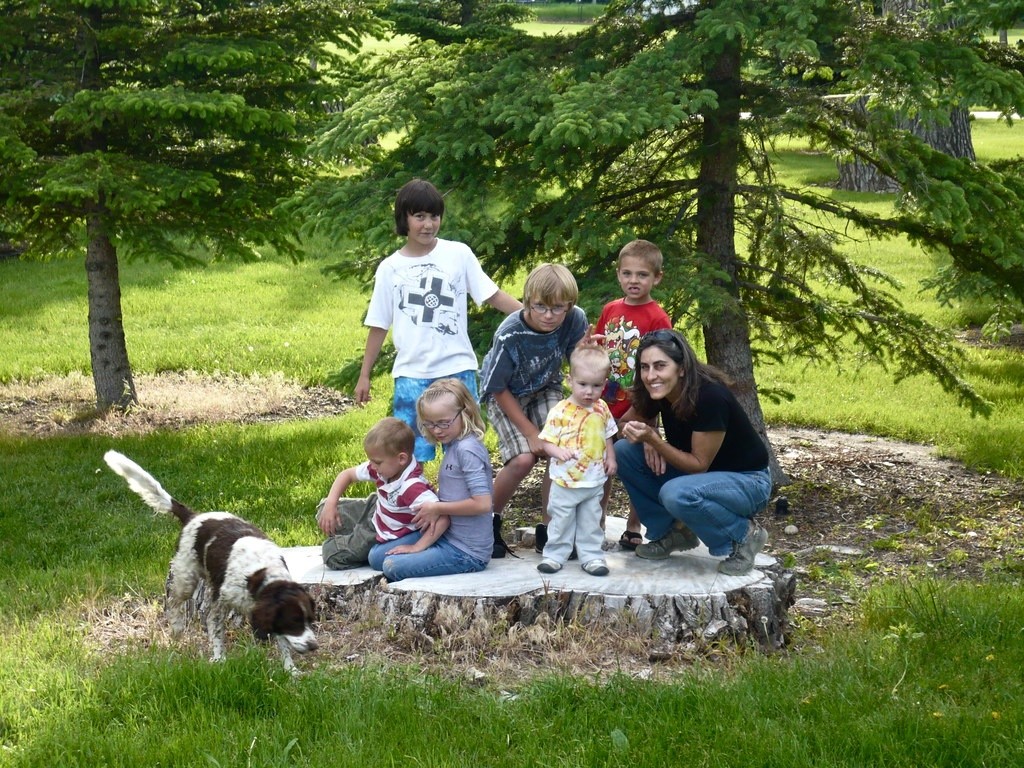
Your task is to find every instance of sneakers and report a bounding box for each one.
[718,519,767,574]
[491,513,523,558]
[636,526,700,560]
[535,523,576,560]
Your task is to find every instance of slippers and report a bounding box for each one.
[619,531,642,548]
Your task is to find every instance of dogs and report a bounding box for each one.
[101,448,324,668]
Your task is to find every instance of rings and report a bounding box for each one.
[624,434,627,439]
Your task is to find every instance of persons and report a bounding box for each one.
[478,264,589,562]
[368,378,493,582]
[595,239,674,552]
[317,417,448,570]
[351,180,522,473]
[611,328,775,577]
[537,346,619,577]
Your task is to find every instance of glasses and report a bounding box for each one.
[529,303,570,316]
[641,330,682,350]
[422,407,466,429]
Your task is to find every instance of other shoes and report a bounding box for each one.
[537,559,563,573]
[581,559,609,576]
